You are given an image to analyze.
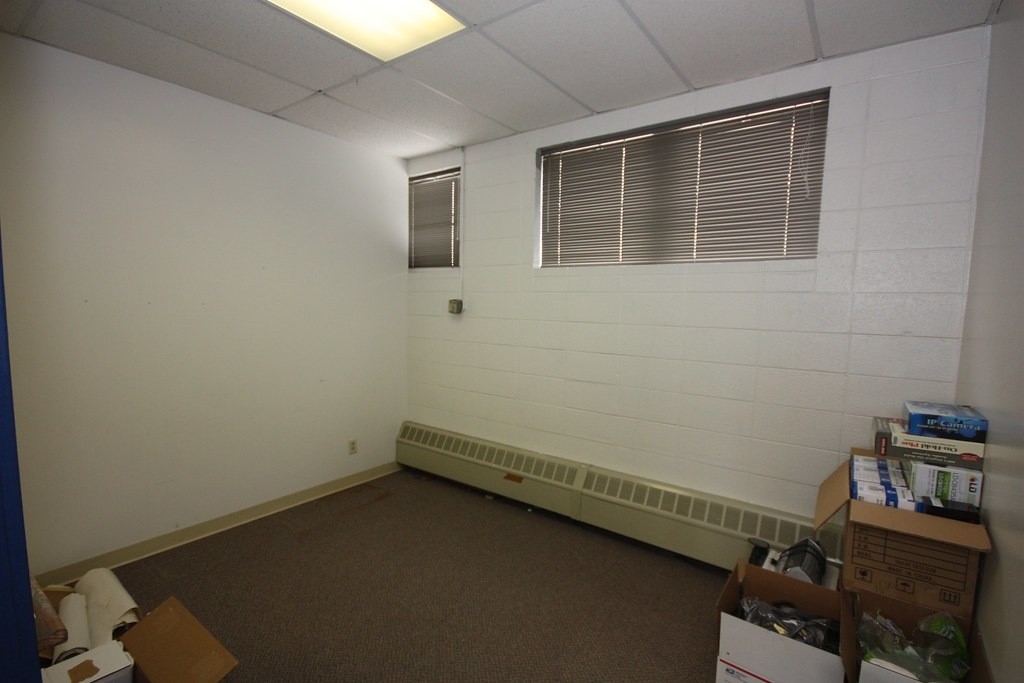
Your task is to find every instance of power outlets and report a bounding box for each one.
[348,438,357,456]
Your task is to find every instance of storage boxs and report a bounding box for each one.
[715,559,846,683]
[839,586,995,683]
[812,446,991,620]
[41,587,237,683]
[850,402,989,524]
